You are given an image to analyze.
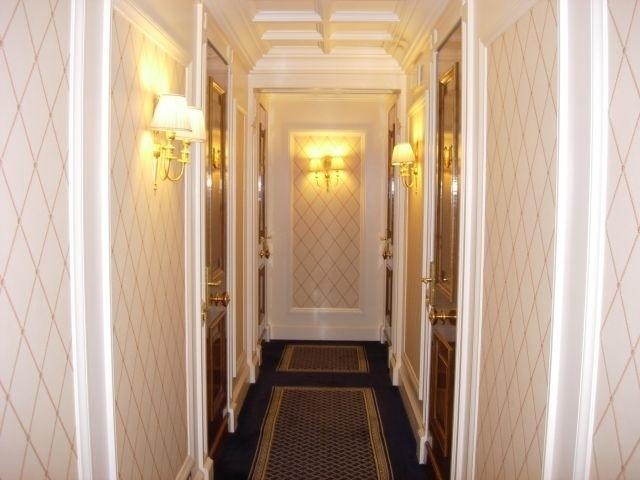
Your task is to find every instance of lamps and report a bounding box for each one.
[388,143,419,194]
[308,154,346,194]
[150,95,207,192]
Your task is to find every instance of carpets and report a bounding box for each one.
[247,346,396,480]
